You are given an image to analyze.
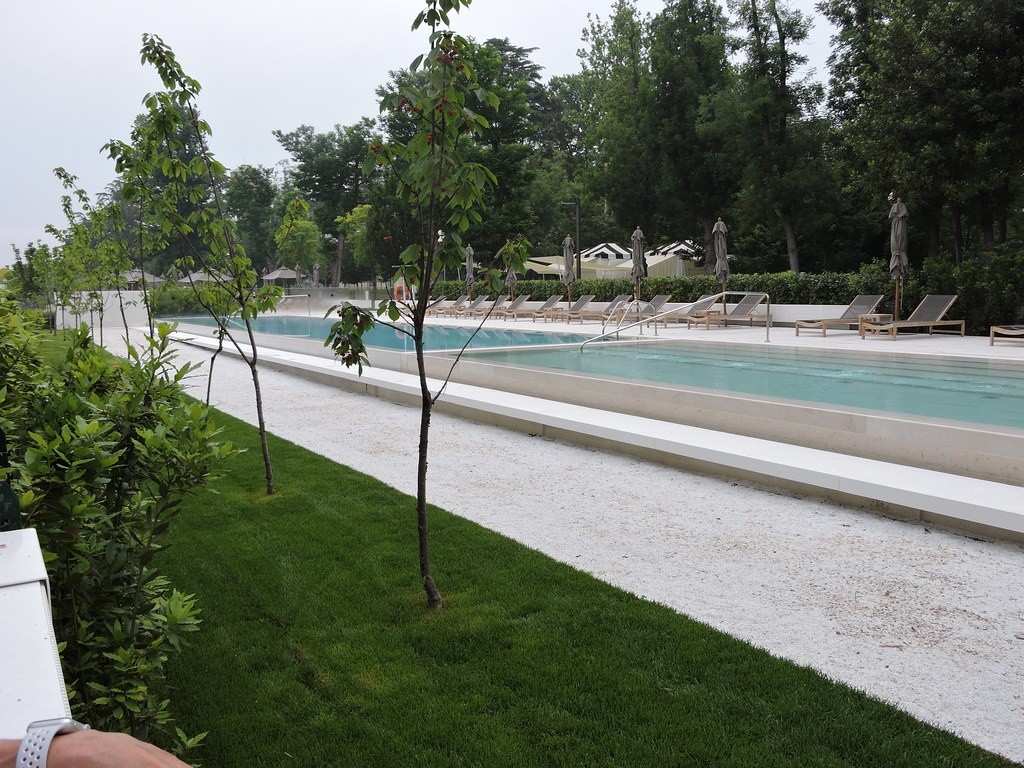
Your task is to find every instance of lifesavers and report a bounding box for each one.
[394,284,408,300]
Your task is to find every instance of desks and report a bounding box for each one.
[859,313,893,335]
[610,307,633,328]
[543,307,564,321]
[695,309,720,329]
[490,305,506,319]
[449,304,467,316]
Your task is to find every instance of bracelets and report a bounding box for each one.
[15,718,90,768]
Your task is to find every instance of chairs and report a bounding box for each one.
[989,325,1024,346]
[859,295,965,342]
[423,292,773,330]
[796,294,884,338]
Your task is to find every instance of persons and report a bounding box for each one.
[0,730,193,768]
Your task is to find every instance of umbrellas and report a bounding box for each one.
[312,264,320,287]
[505,264,517,303]
[110,266,164,291]
[631,226,648,298]
[177,264,233,283]
[712,217,729,298]
[465,243,474,305]
[888,196,910,334]
[561,234,575,309]
[260,265,308,287]
[293,262,302,288]
